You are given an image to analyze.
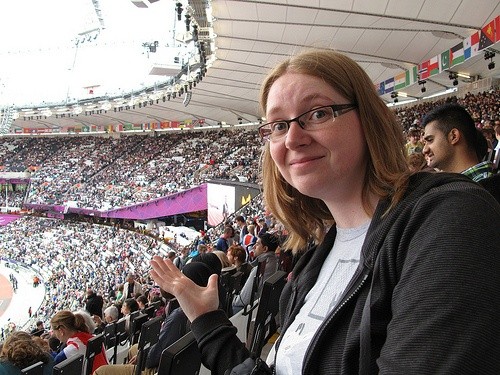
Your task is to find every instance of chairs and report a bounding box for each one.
[0,135,333,375]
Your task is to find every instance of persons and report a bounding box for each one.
[421,103,500,200]
[389,85,500,170]
[0,128,332,375]
[149,49,500,375]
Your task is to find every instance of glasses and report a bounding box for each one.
[257,103,359,142]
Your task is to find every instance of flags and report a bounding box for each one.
[375,14,500,96]
[15,118,206,135]
